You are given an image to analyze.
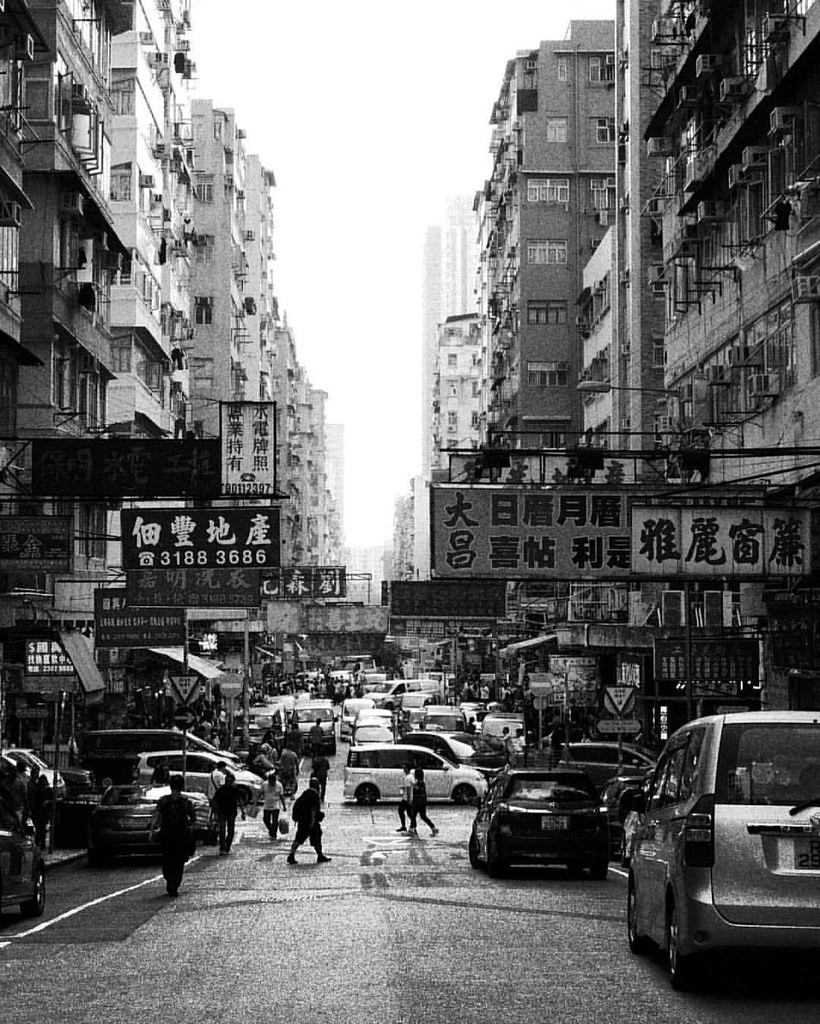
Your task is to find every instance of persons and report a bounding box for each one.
[208,762,244,856]
[0,758,53,851]
[395,764,438,838]
[261,775,286,839]
[287,777,332,863]
[107,662,517,796]
[100,779,118,804]
[150,775,197,897]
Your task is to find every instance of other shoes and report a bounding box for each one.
[431,827,438,836]
[317,857,331,863]
[220,850,226,855]
[226,850,232,854]
[404,831,413,835]
[287,856,297,863]
[397,827,407,831]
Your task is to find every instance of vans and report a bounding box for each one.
[363,679,442,710]
[68,728,242,788]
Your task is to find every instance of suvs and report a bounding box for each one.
[468,763,617,882]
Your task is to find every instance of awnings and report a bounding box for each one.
[499,634,557,660]
[150,646,222,680]
[23,631,105,694]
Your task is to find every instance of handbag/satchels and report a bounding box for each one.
[279,810,289,833]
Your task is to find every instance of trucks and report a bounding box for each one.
[292,701,339,755]
[327,654,388,684]
[418,670,496,695]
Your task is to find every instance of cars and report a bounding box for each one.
[351,720,396,745]
[298,668,325,682]
[0,808,46,921]
[180,791,219,846]
[557,740,676,781]
[90,784,175,864]
[0,748,68,800]
[231,723,264,750]
[130,749,264,806]
[480,713,527,758]
[399,732,510,767]
[625,707,820,986]
[601,774,649,861]
[340,699,376,741]
[233,695,295,748]
[401,693,442,712]
[341,744,489,805]
[403,701,502,733]
[349,709,395,729]
